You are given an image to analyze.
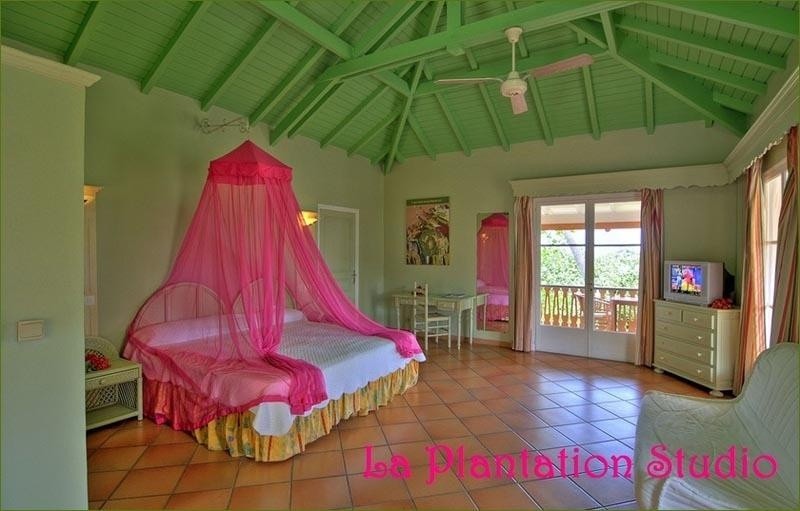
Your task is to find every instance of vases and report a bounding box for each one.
[86,353,110,372]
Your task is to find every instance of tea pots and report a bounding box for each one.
[475,210,516,345]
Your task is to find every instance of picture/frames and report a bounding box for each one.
[476,294,489,334]
[390,290,476,351]
[610,297,640,333]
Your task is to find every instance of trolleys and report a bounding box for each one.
[433,25,596,116]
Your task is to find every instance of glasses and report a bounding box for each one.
[198,110,252,140]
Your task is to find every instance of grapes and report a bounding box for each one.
[85,336,144,434]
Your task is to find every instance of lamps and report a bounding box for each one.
[410,280,451,352]
[573,292,611,332]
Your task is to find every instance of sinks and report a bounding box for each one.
[632,343,799,511]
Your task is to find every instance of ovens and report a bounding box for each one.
[118,274,423,466]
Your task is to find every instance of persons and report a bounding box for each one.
[673,267,699,296]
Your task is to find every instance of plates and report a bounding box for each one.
[0,42,106,511]
[651,297,743,399]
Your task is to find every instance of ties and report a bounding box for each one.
[663,260,735,307]
